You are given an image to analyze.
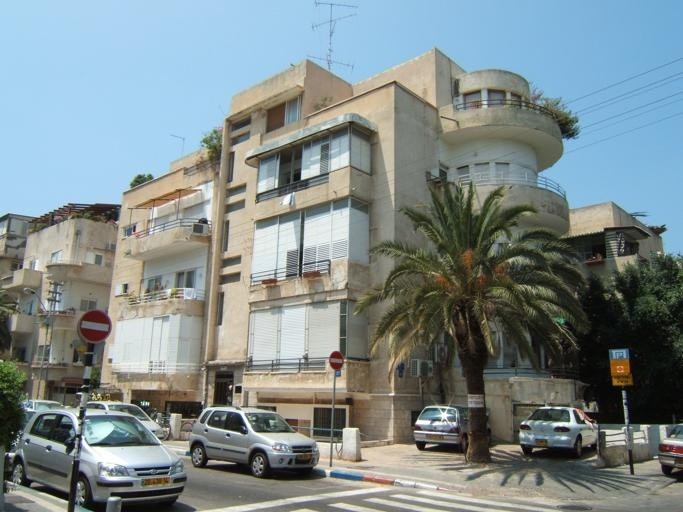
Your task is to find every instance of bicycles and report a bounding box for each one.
[150,408,172,442]
[180,414,196,432]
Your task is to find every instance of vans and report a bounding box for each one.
[11,406,188,512]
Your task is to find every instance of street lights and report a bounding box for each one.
[22,286,51,399]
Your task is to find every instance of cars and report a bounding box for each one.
[657,421,682,476]
[411,402,492,451]
[517,405,598,460]
[5,408,36,472]
[17,399,72,435]
[187,403,320,479]
[77,400,163,439]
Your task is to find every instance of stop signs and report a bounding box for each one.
[328,350,345,371]
[76,310,112,345]
